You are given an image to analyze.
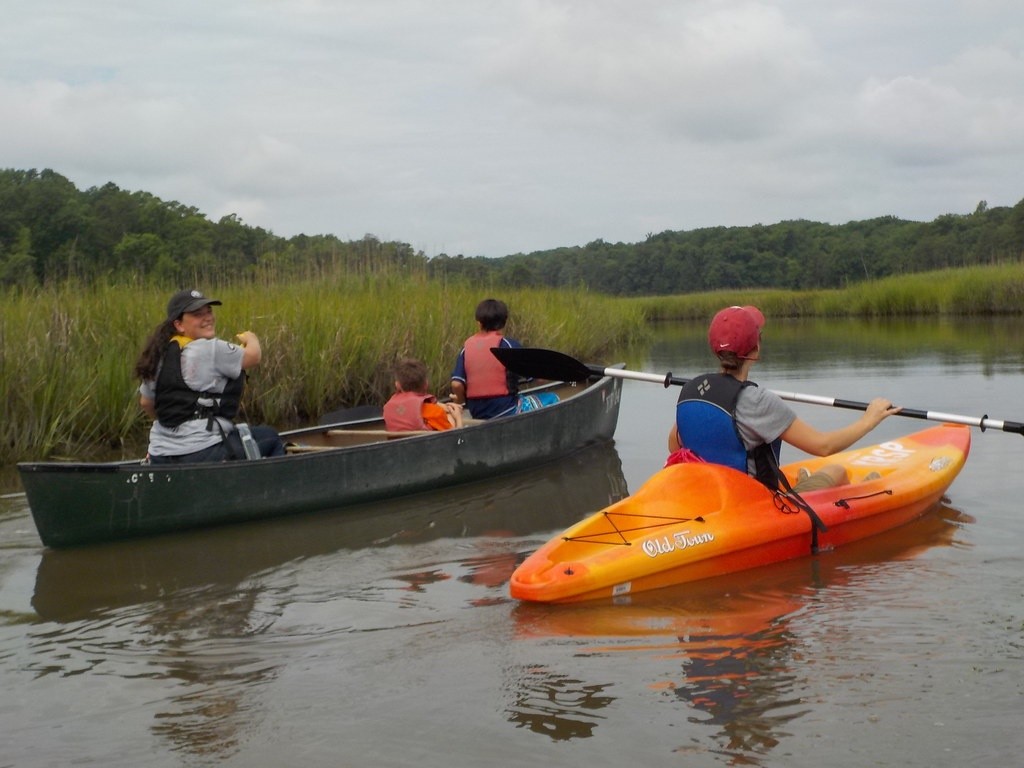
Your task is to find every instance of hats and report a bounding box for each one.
[166,289,222,323]
[705,306,766,361]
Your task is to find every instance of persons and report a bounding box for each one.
[384,362,463,434]
[668,304,907,501]
[140,289,285,462]
[450,299,560,421]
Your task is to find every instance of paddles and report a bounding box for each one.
[489,345,1024,438]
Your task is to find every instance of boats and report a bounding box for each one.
[16,356,626,547]
[509,419,971,603]
